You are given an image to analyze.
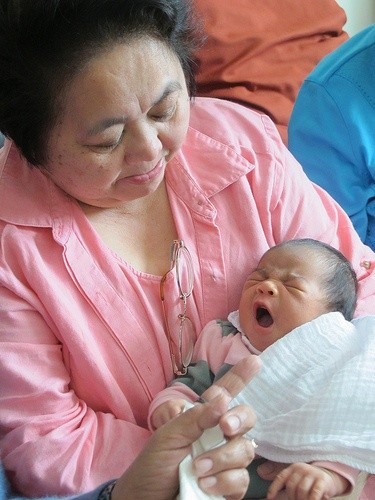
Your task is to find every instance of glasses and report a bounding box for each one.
[158,239,197,376]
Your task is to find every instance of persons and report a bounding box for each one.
[287,18,374,247]
[148,239,362,500]
[0,353,262,500]
[0,1,374,496]
